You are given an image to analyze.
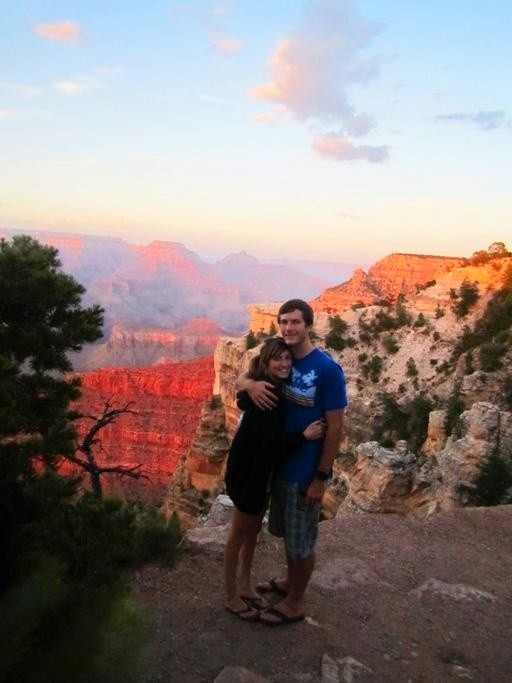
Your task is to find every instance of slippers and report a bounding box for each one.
[258,604,308,626]
[240,593,269,610]
[223,597,260,622]
[253,577,288,595]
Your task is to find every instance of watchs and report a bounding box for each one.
[313,468,330,481]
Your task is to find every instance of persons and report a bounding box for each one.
[222,339,329,623]
[233,297,350,626]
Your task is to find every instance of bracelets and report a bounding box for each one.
[243,377,254,391]
